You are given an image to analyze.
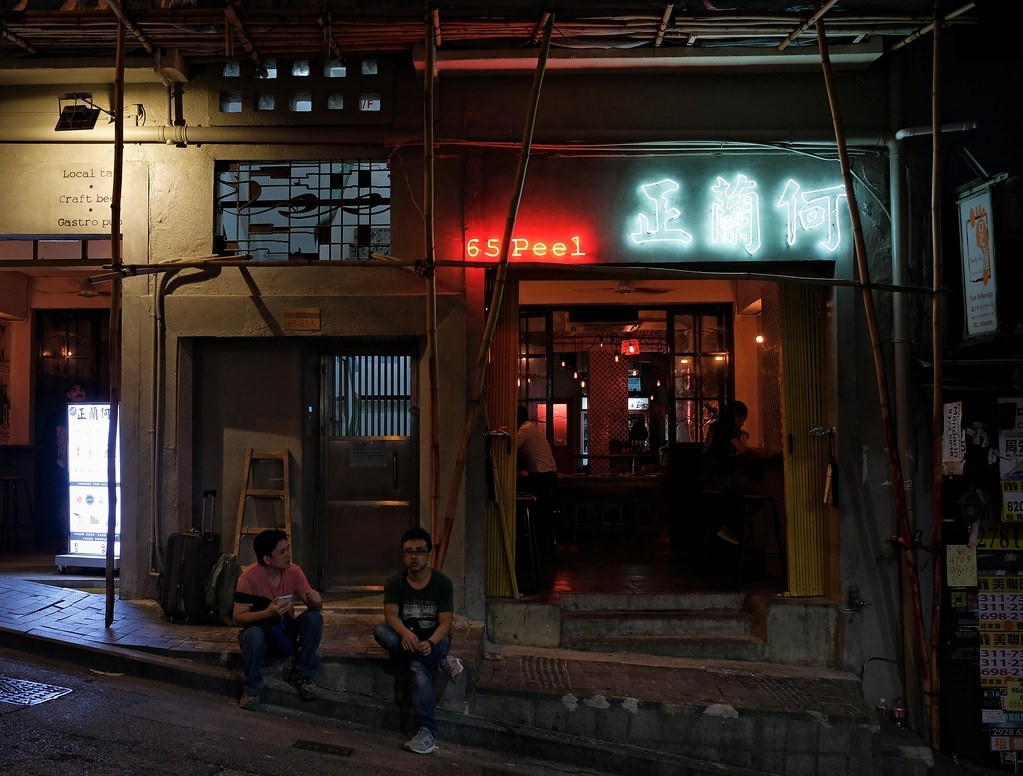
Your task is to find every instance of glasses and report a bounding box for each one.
[402,550,429,557]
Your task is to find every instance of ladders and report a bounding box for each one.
[233,445,292,572]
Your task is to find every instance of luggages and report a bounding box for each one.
[156,488,220,627]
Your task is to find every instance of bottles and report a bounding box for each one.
[877,698,887,721]
[906,699,916,733]
[893,695,907,723]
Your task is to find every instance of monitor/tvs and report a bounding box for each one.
[628,397,649,411]
[628,376,642,392]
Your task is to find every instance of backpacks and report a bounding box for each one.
[202,551,243,627]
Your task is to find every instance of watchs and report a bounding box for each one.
[426,639,434,653]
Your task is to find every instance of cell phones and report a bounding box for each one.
[277,595,293,605]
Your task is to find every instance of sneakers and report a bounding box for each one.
[240,692,260,710]
[440,654,464,685]
[289,675,320,699]
[404,727,436,754]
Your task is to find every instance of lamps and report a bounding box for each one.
[54,91,117,133]
[517,321,764,391]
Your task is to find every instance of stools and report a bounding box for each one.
[516,488,785,594]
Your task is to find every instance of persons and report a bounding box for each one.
[516,405,558,506]
[373,527,455,752]
[700,400,749,544]
[232,527,323,707]
[45,375,90,553]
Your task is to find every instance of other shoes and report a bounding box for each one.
[718,528,739,545]
